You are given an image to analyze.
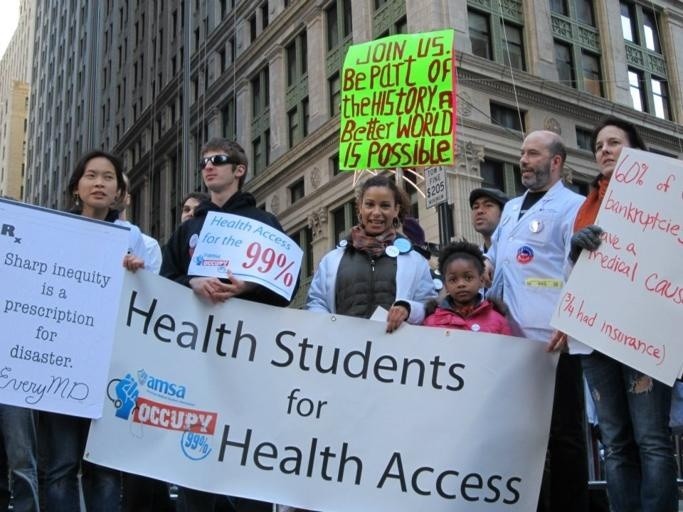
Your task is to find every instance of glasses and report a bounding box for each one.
[199,153,242,170]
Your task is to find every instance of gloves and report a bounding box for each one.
[567,224,603,260]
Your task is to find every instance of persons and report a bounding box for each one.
[1,138,302,510]
[305,117,683,511]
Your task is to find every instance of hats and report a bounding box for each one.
[469,185,510,205]
